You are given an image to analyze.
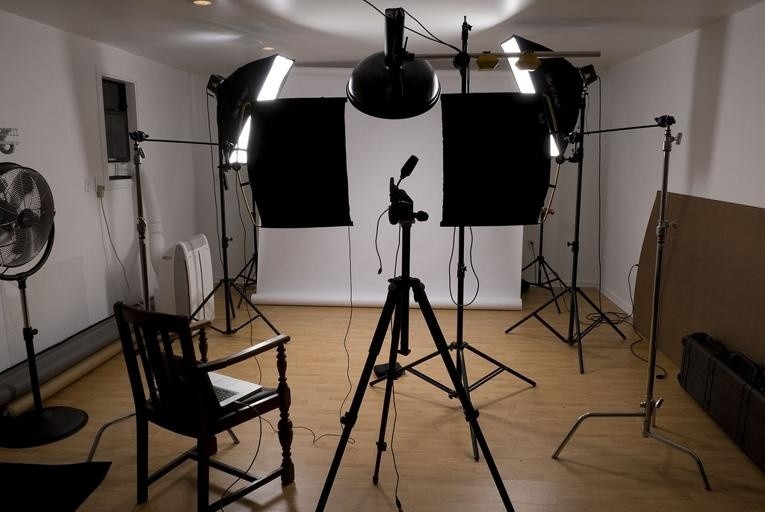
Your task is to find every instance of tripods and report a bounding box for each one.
[522,202,571,314]
[164,143,282,348]
[314,225,517,512]
[230,192,258,309]
[504,97,627,373]
[85,145,240,466]
[550,127,716,491]
[369,227,537,462]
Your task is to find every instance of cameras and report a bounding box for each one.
[374,154,429,275]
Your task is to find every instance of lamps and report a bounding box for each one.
[345,8,445,122]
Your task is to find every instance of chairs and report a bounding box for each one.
[113,301,295,512]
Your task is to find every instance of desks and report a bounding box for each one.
[0,460,113,511]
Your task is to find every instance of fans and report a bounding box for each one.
[0,161,89,449]
